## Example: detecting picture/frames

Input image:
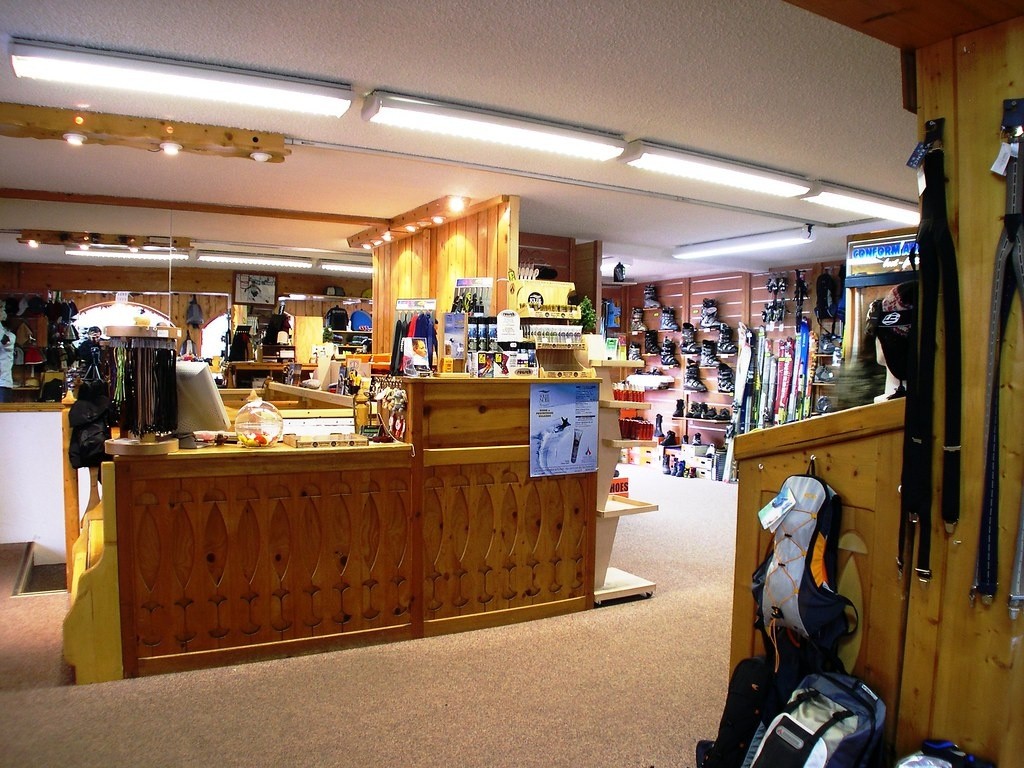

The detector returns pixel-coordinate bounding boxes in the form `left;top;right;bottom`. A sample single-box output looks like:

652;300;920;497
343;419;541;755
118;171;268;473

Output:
232;270;278;309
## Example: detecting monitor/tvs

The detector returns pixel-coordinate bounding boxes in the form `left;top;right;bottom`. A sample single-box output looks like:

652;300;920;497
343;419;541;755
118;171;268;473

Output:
173;361;231;448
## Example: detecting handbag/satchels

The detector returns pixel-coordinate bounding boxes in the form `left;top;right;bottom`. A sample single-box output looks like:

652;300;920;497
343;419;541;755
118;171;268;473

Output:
751;459;858;673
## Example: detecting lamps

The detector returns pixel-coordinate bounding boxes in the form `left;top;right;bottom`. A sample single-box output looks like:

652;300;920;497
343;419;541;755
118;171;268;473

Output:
9;36;351;121
61;133;90;146
129;246;142;253
360;88;623;162
250;152;272;163
28;241;41;249
799;180;920;225
78;243;90;250
158;142;182;157
362;216;445;250
672;225;816;259
617;139;811;200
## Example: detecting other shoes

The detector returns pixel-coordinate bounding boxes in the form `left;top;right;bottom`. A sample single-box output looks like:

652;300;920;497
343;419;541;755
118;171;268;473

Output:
662;454;696;478
692;433;701;445
685;402;730;420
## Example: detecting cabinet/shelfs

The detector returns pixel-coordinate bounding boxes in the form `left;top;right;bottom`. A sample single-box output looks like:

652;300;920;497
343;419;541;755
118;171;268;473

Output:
628;328;836;423
588;359;657;604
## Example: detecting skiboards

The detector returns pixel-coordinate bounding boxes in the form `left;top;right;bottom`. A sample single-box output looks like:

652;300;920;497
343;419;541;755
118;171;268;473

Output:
720;317;820;484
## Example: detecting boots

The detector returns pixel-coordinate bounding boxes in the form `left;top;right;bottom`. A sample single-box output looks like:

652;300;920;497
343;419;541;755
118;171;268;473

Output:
700;339;721;367
660;307;679;330
660;430;676;446
645;330;662;354
630;307;648;331
700;298;721;328
717;363;735;393
717;324;738;353
628;344;642;361
660;339;679;365
654;414;665;437
644;283;661;308
680;323;701;353
673;399;684;417
684;358;707;391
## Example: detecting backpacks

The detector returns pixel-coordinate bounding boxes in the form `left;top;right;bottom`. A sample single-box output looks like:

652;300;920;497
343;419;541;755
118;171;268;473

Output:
814;269;841;339
739;668;887;768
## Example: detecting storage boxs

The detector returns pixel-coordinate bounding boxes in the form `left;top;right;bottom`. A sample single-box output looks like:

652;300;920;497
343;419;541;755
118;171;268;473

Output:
621;438;736;483
508;279;575;312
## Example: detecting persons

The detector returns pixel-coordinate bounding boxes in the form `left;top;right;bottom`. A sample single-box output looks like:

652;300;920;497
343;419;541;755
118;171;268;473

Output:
77;326;108;378
411;339;427;365
0;324;16;402
